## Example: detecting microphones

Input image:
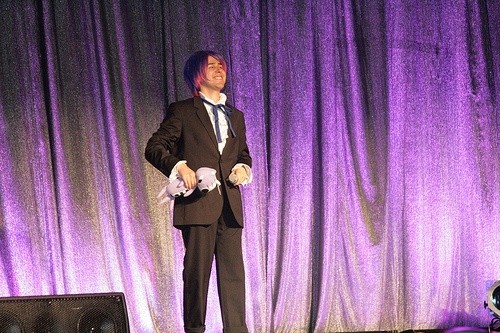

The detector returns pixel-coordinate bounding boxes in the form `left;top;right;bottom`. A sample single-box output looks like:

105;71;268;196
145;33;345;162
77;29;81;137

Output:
229;173;238;184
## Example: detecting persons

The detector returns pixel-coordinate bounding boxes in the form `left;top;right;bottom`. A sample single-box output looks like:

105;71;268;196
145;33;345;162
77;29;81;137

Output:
144;51;253;333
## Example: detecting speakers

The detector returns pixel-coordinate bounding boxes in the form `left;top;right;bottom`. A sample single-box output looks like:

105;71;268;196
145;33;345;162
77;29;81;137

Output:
0;292;130;333
